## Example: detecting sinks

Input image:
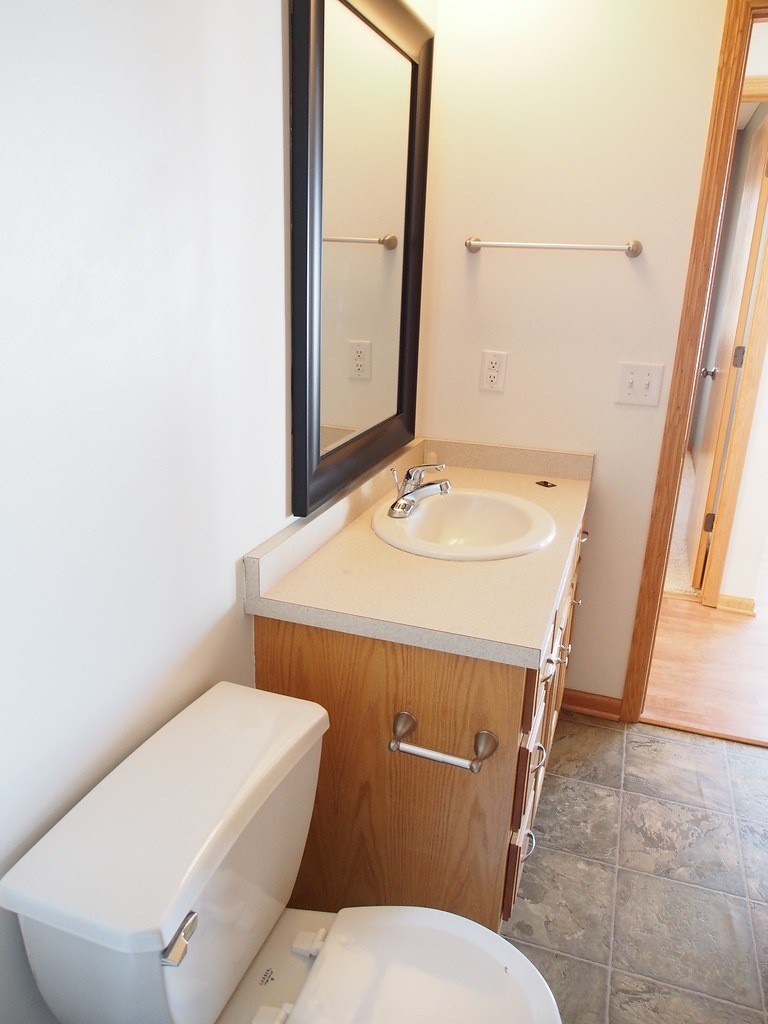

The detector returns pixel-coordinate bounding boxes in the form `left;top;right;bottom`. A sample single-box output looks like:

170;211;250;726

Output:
370;490;558;563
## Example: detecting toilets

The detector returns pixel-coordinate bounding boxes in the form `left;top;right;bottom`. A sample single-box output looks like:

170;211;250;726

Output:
3;680;562;1024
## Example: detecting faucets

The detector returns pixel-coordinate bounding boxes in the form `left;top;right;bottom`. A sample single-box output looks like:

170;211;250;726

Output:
387;462;453;518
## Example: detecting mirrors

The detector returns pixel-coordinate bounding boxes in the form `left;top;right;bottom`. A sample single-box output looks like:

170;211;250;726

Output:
293;0;434;516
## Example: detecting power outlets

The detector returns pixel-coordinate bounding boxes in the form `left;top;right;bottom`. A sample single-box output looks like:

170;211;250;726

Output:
479;349;507;392
348;341;372;379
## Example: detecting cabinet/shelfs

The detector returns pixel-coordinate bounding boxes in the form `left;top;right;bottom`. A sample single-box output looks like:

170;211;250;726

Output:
253;515;588;934
